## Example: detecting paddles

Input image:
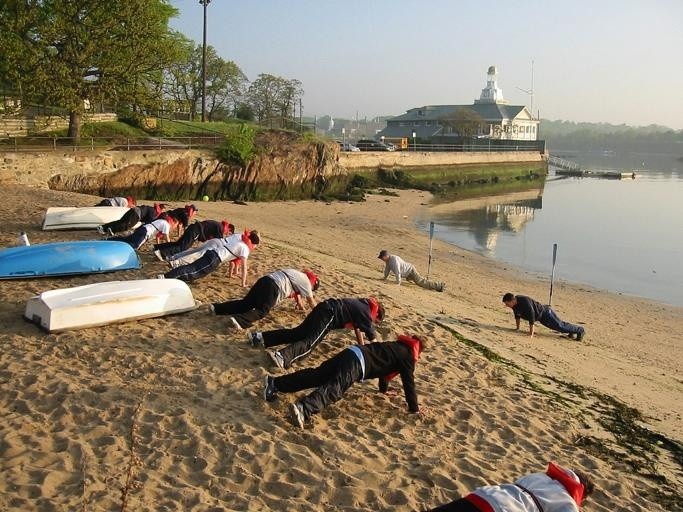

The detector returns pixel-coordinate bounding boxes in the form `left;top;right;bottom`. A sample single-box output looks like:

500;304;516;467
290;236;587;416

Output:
548;244;557;304
428;222;434;270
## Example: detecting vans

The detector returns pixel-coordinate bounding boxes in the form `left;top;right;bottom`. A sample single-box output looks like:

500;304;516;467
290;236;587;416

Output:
355;138;395;152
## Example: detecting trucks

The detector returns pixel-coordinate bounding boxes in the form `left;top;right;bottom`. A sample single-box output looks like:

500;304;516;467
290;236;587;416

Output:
378;136;408;151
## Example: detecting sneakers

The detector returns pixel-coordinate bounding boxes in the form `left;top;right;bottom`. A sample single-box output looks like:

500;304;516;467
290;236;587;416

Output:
289;400;306;429
568;326;585;341
377;250;391;258
230;316;242;329
440;281;446;292
97;225;179;279
267;351;284;368
207;303;216;313
264;373;277;403
247;332;260;347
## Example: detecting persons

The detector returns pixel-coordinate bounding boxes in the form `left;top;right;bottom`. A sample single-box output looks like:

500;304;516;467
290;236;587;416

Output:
209;269;319;331
83;97;91;113
94;196;260;288
263;335;429;430
248;297;385;371
429;462;593;512
378;250;445;292
502;293;586;341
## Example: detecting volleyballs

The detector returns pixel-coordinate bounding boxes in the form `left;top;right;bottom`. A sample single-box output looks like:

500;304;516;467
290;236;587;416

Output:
203;195;209;201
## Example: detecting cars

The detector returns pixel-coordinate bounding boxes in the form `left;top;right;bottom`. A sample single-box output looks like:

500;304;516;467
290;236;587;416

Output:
339;143;361;152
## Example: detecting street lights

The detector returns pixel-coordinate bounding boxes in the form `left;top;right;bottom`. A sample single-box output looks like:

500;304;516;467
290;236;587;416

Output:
199;0;212;121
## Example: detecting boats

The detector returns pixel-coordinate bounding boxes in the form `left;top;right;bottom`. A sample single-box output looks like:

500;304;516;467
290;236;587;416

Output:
0;241;141;282
41;205;142;231
22;278;199;335
